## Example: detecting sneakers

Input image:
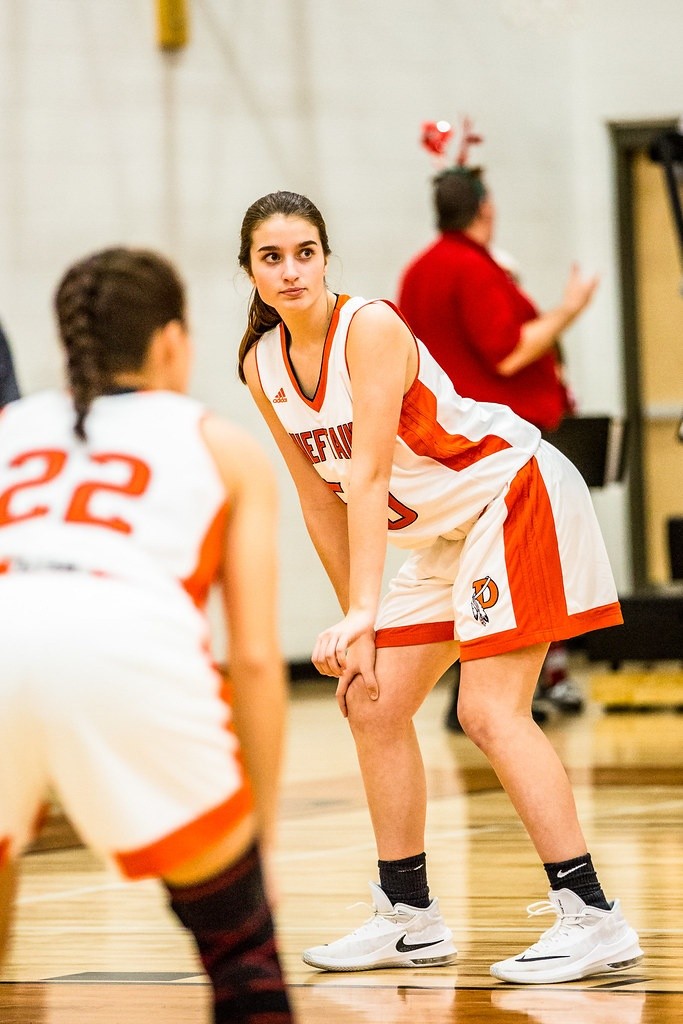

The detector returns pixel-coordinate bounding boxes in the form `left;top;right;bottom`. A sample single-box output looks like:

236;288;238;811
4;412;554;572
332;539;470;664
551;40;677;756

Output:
301;885;458;969
488;889;644;983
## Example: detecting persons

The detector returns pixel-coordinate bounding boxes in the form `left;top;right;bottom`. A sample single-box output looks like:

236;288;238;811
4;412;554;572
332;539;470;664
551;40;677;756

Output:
237;190;645;984
396;167;604;735
0;246;298;1024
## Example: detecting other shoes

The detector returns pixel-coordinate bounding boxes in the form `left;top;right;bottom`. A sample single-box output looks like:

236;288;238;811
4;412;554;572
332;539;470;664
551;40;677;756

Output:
449;653;583;732
